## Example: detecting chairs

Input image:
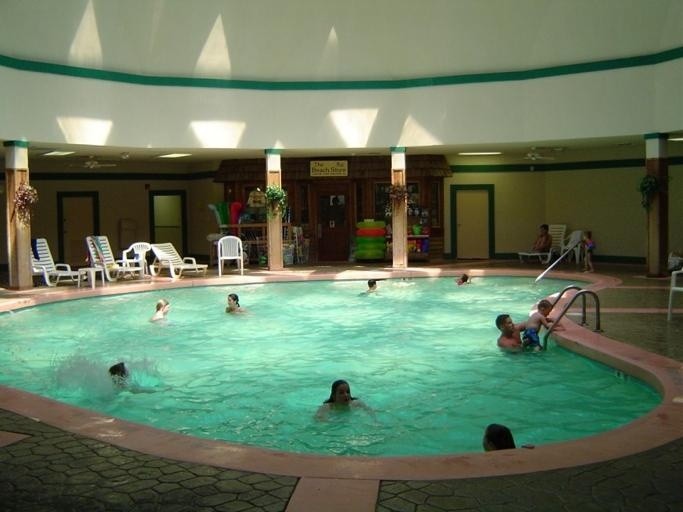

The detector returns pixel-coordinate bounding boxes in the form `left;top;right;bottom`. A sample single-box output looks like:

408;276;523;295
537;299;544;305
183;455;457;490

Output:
205;232;222;266
559;228;587;262
667;263;683;320
28;235;245;287
515;224;567;261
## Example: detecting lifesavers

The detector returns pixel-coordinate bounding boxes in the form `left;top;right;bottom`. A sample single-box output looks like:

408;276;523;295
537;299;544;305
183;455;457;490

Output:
354;220;386;260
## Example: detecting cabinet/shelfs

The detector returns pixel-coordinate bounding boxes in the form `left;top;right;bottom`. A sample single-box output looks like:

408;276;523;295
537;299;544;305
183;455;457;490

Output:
216;224;297;267
382;232;431;261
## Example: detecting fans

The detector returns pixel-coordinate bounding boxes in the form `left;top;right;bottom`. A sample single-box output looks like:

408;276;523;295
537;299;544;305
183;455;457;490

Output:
64;155;117;169
508;146;557;163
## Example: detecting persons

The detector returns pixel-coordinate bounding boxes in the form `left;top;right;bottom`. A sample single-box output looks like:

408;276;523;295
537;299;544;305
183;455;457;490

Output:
521;299;566;353
454;273;472;286
363;278;380;294
532;223;552;253
312;378;378;424
580;229;596;274
108;362;130;385
225;293;249;315
481;422;535;451
150;298;170;322
494;312;556;350
